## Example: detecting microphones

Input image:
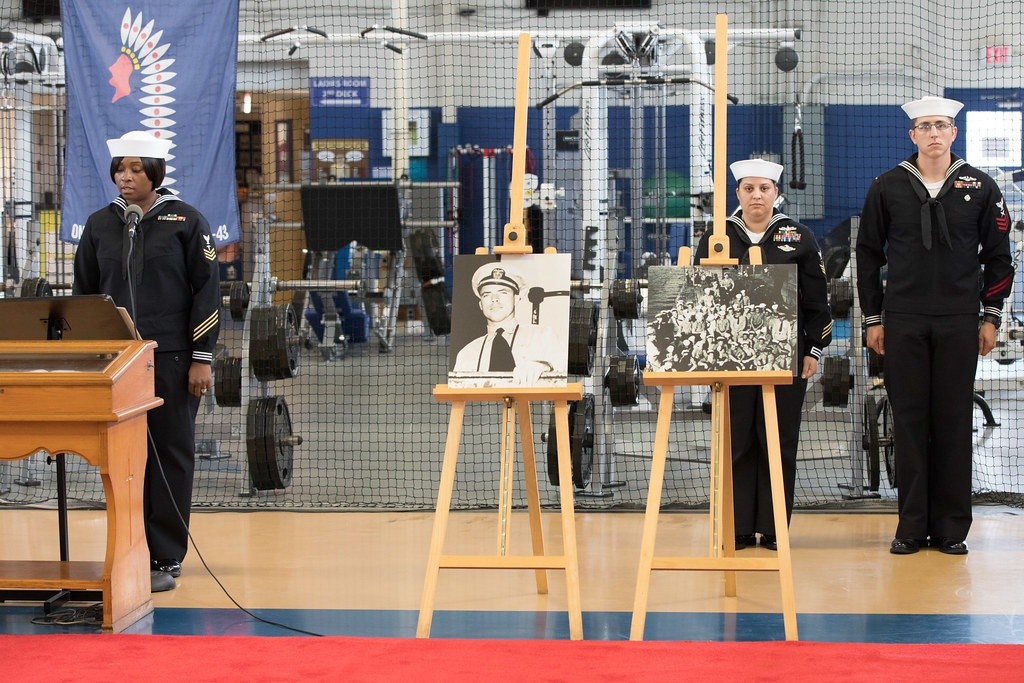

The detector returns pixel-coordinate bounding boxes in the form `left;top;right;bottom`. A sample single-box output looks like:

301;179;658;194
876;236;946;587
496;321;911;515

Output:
124;204;143;238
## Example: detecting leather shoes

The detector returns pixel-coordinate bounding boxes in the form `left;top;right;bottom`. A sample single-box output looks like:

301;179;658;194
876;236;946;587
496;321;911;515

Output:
890;536;923;554
938;538;968;554
151;558;182;577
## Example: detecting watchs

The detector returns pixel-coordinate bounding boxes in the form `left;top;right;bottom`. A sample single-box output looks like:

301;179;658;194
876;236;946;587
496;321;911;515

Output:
983;314;1001;329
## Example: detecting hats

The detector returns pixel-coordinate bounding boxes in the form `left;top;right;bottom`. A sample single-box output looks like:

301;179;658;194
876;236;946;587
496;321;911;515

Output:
470;261;524;295
900;96;964;119
729;159;784;184
105;130;172;158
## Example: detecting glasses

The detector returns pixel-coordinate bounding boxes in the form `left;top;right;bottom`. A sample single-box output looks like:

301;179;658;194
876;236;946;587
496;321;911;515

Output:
913;121;955;130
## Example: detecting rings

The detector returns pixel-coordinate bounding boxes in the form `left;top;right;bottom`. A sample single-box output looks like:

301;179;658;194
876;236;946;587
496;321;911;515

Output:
201;388;207;394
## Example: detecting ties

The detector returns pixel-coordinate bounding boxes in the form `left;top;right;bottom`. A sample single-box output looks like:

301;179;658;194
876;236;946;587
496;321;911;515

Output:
489;327;515;371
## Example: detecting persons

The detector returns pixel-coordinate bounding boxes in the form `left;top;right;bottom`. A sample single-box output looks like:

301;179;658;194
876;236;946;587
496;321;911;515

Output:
453;263;561;387
71;130;222;576
329;155;351;178
692;159;835;550
649;270;796;373
855;97;1016;555
239;167;260;199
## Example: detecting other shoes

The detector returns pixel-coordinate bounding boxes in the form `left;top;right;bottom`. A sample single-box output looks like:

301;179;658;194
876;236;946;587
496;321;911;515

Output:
735;531;756;550
759;534;778;550
